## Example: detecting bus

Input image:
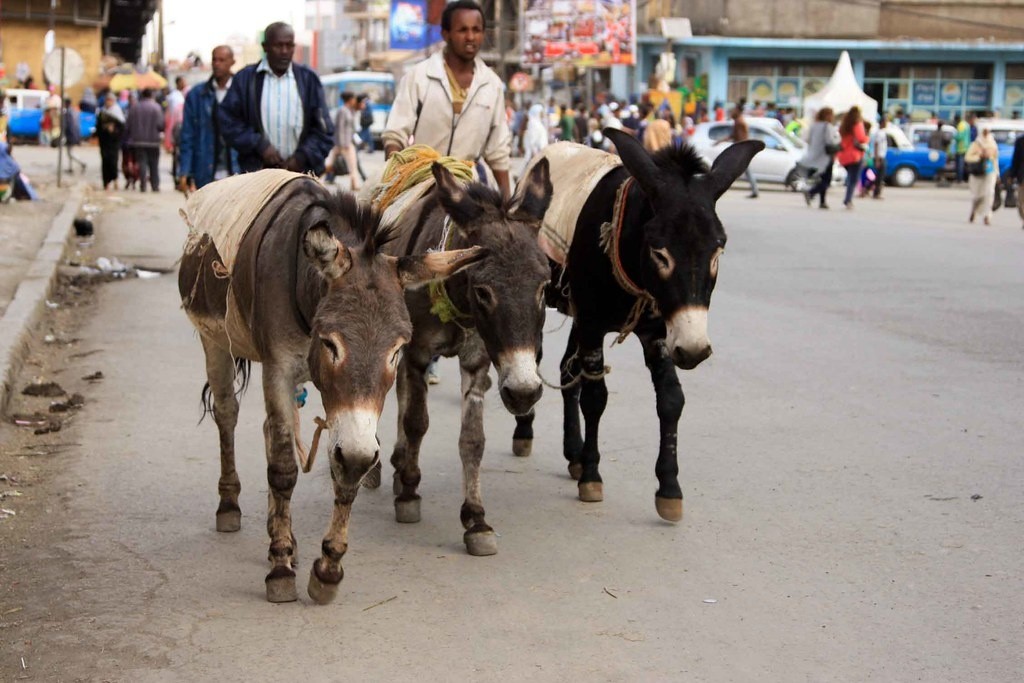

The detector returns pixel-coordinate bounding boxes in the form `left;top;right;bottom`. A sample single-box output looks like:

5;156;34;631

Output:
318;70;399;149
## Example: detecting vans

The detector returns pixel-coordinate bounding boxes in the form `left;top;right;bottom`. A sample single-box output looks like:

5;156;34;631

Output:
0;88;52;120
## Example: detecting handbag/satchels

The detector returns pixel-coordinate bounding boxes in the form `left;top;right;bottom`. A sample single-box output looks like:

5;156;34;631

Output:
360;115;373;127
1008;186;1016;209
992;185;1002;212
824;144;842;154
853;138;869;151
333;155;348;176
965;157;987;177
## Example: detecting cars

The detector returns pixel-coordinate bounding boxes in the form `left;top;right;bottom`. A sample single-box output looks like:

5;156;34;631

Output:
878;119;1024;189
682;122;848;192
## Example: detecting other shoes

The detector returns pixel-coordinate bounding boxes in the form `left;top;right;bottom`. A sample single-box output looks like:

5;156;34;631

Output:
804;191;810;205
820;204;828;208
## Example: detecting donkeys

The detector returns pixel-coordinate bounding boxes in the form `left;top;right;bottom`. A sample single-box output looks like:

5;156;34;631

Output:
178;126;766;604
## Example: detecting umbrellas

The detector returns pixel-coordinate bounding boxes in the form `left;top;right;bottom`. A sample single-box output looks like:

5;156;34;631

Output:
109;67;167;106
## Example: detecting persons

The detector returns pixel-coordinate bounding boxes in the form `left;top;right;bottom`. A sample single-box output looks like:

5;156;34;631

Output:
663;108;674;128
806;106;886;209
588;92;655;154
79;86;169;190
180;46;238;196
0;77;61;199
65;99;85;174
384;0;511;385
507;91;586;156
701;97;801;199
166;77;186;185
892;112;910;125
927;112;1024;228
326;94;366;182
324;91;361;189
360;94;374;153
217;22;333;174
681;118;693;129
